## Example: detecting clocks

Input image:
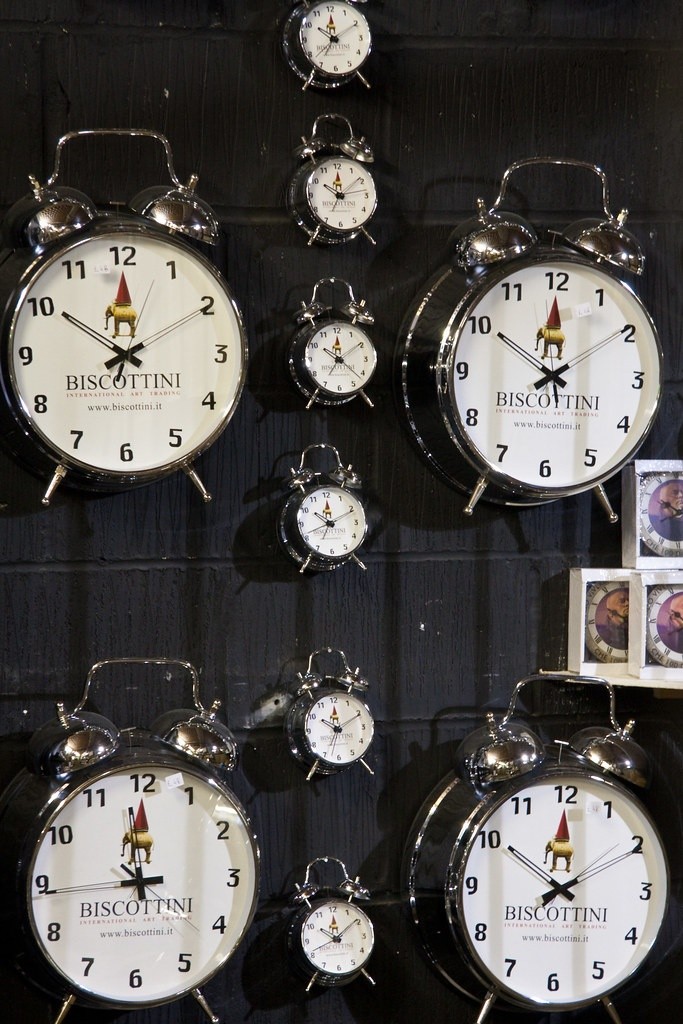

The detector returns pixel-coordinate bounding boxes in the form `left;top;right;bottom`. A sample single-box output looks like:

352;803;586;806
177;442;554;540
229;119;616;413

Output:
390;156;659;523
402;674;673;1024
0;128;250;503
274;442;370;574
284;113;379;245
288;277;378;410
285;856;377;991
0;656;261;1024
278;0;374;94
628;571;683;679
567;567;679;676
621;459;683;570
283;648;377;782
